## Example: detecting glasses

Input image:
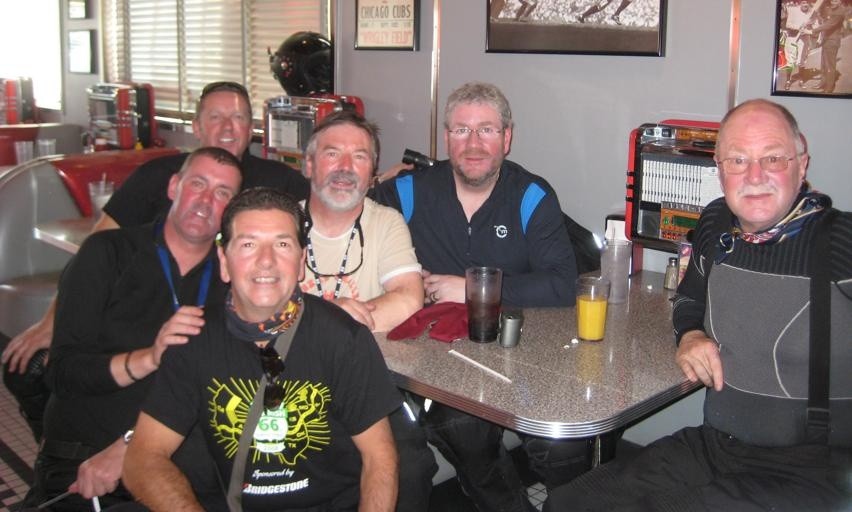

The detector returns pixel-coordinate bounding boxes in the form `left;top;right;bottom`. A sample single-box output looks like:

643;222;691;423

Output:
447;126;505;135
717;155;800;174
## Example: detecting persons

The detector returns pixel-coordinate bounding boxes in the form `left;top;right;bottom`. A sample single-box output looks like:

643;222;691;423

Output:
295;111;425;340
2;80;417;443
120;184;404;511
578;0;635;29
513;0;541;25
18;143;244;512
366;82;594;512
810;0;849;96
784;1;816;90
541;96;852;512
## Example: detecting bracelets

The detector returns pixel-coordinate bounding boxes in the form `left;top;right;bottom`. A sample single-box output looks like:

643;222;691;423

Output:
373;176;381;187
125;348;140;383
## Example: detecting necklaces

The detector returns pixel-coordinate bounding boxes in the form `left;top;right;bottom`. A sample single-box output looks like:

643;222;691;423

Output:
301;197;365;277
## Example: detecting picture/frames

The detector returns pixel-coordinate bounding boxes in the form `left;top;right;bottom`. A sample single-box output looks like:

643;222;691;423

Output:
67;0;94;74
354;0;420;51
770;0;852;99
485;0;667;57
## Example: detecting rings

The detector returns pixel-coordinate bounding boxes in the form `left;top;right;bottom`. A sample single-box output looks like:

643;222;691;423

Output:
430;290;437;305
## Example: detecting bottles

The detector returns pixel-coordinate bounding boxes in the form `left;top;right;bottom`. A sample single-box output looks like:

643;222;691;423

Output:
81;132;143;153
664;258;678;291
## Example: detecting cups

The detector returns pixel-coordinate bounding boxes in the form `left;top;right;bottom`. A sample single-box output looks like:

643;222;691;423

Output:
574;276;611;342
500;311;523;348
14;138;56;164
600;239;632;304
465;267;503;343
89;181;115;223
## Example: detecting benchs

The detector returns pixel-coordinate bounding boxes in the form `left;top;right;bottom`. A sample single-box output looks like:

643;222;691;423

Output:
1;148;196;342
